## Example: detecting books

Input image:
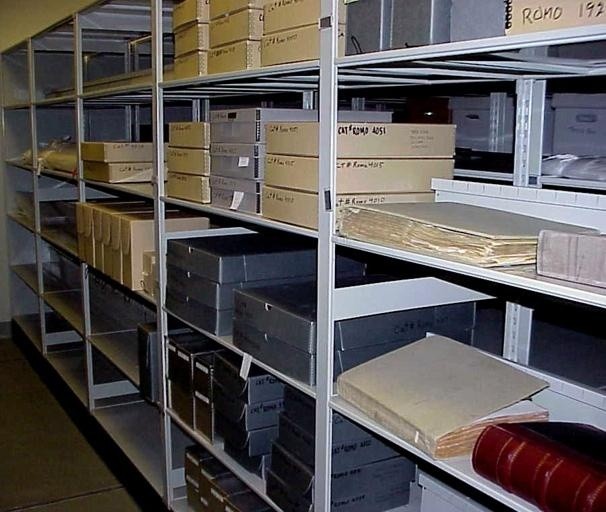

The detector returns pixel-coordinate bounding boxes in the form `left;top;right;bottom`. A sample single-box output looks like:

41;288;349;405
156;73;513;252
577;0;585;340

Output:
335;201;600;269
473;423;606;512
337;335;550;462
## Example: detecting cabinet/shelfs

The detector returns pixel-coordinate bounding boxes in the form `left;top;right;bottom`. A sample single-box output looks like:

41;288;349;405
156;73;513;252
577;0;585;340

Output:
30;5;198;434
153;6;526;511
0;2;605;512
74;3;365;512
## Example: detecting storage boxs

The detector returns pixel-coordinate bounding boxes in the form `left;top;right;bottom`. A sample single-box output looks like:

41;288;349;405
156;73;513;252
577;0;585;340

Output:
315;5;606;512
449;93;604;160
504;1;605;35
172;2;346;78
80;108;456;232
76;200;367;338
136;273;489;512
